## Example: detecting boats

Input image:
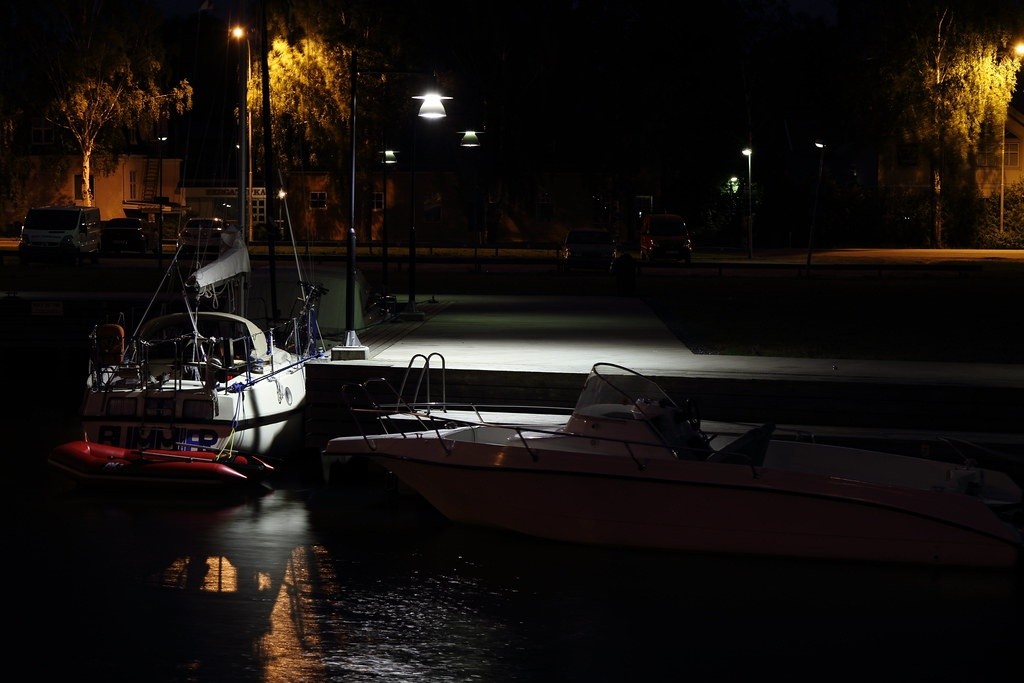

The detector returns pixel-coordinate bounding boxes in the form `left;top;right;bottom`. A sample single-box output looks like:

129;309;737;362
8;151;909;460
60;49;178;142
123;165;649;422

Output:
320;361;1023;578
72;213;341;458
349;351;1024;485
46;436;280;492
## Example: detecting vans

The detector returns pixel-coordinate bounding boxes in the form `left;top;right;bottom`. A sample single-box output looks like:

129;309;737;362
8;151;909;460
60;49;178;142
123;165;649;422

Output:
17;204;101;262
636;213;695;264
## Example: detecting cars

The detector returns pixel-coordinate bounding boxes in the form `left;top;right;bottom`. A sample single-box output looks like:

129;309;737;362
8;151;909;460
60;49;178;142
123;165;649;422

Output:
174;216;231;256
100;214;159;256
560;225;617;272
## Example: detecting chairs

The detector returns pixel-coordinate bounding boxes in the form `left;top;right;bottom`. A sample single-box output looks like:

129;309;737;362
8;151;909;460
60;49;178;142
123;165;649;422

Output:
708;423;776;464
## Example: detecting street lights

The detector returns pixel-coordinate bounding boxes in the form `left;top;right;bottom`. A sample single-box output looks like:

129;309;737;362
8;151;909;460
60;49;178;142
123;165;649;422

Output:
229;22;256;244
156;132;168;256
743;143;754;259
456;98;486;271
377;123;401;303
405;72;455;314
997;37;1024;234
805;137;828;264
276;186;287;229
